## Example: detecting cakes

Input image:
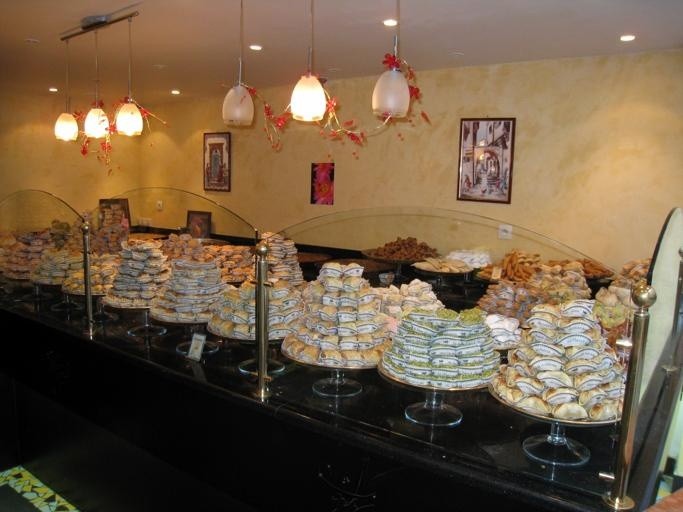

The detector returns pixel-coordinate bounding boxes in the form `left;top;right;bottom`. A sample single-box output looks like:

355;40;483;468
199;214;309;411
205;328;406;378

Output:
380;306;502;390
103;238;170;309
147;256;227;323
1;222;255;297
367;236;653;348
491;299;627;422
280;263;389;370
208;278;301;342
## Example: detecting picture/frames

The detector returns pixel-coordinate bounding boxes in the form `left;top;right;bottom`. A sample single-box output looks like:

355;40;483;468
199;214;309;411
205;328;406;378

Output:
454;116;516;204
202;131;231;192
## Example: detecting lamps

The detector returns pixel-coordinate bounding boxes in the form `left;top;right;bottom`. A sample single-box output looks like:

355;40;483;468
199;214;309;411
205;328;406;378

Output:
52;10;146;143
219;1;410;128
